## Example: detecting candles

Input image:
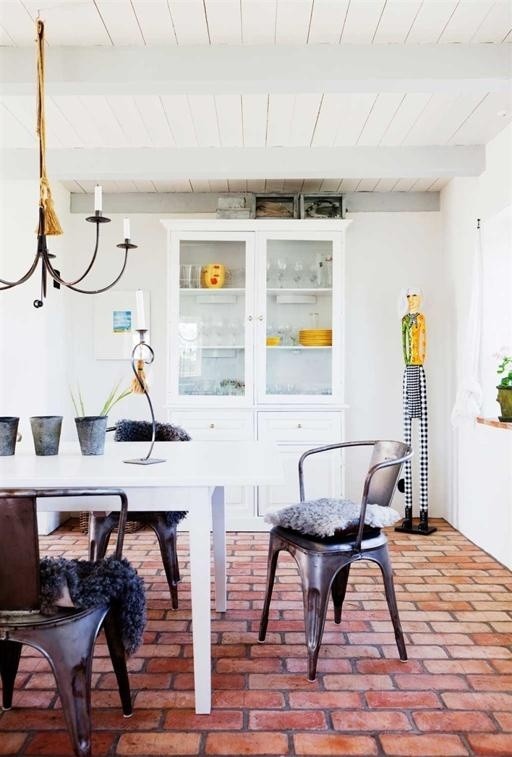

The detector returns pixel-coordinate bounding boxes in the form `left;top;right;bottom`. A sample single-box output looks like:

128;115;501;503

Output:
135;286;146;329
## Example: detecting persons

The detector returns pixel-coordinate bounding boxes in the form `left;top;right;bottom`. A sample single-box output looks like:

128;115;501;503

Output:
399;285;429;530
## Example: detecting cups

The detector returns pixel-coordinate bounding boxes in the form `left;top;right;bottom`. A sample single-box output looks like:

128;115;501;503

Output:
0;416;20;456
265;380;333;395
179;379;243;396
179;262;203;289
75;414;109;455
30;414;62;456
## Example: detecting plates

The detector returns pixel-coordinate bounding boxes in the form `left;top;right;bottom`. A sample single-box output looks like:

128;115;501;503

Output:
298;328;334;347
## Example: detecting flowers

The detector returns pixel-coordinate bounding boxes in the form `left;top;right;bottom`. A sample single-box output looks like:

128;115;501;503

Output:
496;355;512;387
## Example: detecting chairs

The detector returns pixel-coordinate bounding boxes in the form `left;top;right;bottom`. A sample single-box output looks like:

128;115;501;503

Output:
258;438;416;683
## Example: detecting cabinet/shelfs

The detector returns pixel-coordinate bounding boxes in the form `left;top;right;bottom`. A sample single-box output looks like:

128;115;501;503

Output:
165;230;351;532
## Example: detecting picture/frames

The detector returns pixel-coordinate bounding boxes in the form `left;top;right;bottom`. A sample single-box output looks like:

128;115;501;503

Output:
252;192;346;219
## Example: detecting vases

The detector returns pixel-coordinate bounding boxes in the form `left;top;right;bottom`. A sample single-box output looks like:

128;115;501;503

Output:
494;385;512;423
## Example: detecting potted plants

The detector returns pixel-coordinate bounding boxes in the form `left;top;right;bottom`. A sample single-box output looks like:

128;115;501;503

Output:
67;379;134;457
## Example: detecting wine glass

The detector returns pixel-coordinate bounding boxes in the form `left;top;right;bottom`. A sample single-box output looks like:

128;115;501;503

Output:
266;253;332;288
266;321;301;345
177;317;244;348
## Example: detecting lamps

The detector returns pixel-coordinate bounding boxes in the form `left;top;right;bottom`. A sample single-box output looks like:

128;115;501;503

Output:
2;15;140;308
